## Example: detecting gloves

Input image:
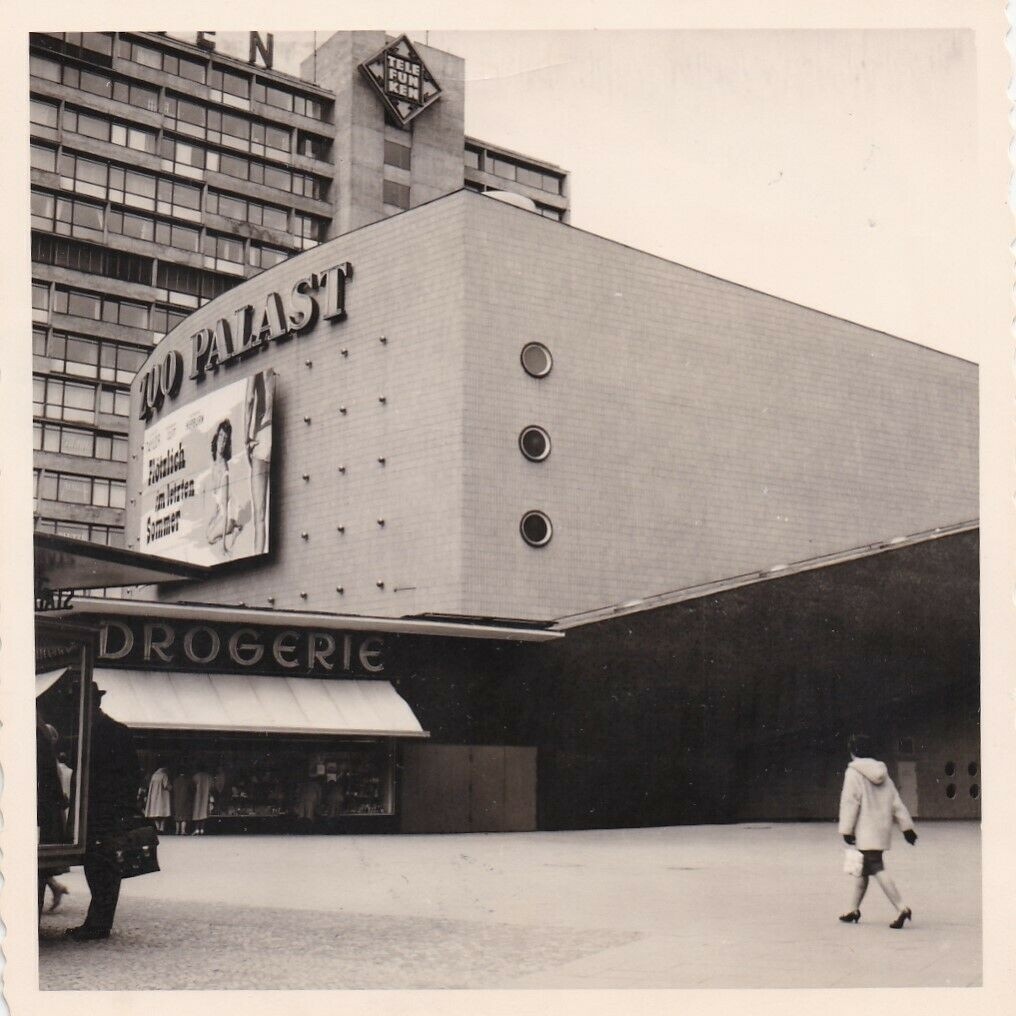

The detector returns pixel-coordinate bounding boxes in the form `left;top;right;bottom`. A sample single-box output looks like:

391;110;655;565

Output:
903;829;917;845
843;834;856;845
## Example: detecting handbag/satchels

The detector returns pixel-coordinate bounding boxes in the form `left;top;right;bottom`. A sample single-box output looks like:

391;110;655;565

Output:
839;847;864;877
108;825;160;879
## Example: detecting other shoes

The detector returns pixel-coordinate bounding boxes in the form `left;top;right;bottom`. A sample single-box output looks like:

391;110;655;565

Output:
180;831;189;835
175;831;179;835
156;830;163;835
65;925;111;941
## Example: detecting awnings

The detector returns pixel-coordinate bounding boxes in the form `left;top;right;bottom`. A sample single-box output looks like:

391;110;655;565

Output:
91;669;429;737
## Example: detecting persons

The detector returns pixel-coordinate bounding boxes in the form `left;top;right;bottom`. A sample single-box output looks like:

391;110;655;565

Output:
42;702;139;940
839;735;918;929
144;761;211;836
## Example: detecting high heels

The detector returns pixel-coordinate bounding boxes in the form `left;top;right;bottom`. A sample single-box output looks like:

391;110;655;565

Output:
192;829;199;836
200;828;204;835
48;884;69;912
889;906;911;929
839;909;860;924
